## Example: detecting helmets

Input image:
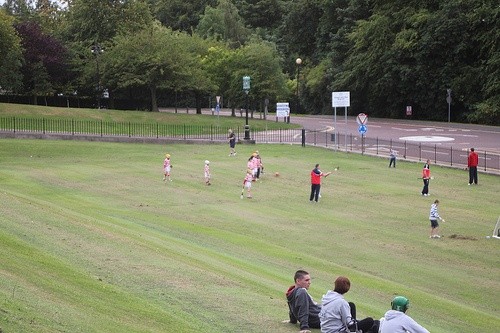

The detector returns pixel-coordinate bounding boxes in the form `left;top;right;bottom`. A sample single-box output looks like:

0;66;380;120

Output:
390;296;410;314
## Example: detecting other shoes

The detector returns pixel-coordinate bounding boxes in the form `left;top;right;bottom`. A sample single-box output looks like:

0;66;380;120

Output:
247;195;252;197
206;181;211;184
468;181;476;184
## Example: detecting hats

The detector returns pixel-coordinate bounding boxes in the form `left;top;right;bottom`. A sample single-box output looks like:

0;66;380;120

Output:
205;159;209;164
256;150;259;155
253;152;257;156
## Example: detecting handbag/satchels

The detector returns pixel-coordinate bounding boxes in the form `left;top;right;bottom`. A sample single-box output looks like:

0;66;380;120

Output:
336;316;363;332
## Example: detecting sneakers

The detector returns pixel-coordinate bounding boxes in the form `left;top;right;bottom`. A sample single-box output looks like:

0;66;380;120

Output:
430;234;440;238
234;151;237;155
422;192;430;196
228;154;232;156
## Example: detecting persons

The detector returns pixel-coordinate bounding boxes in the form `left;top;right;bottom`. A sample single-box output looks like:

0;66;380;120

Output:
205;160;212;185
240;151;264;199
162;153;173;182
378;296;430;333
318;276;374;333
389;148;396;168
309;164;327;203
468;148;479;185
420;160;431;196
228;128;237;157
282;270;356;333
429;200;445;238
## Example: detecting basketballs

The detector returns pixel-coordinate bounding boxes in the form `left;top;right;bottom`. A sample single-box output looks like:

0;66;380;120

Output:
275;171;280;179
